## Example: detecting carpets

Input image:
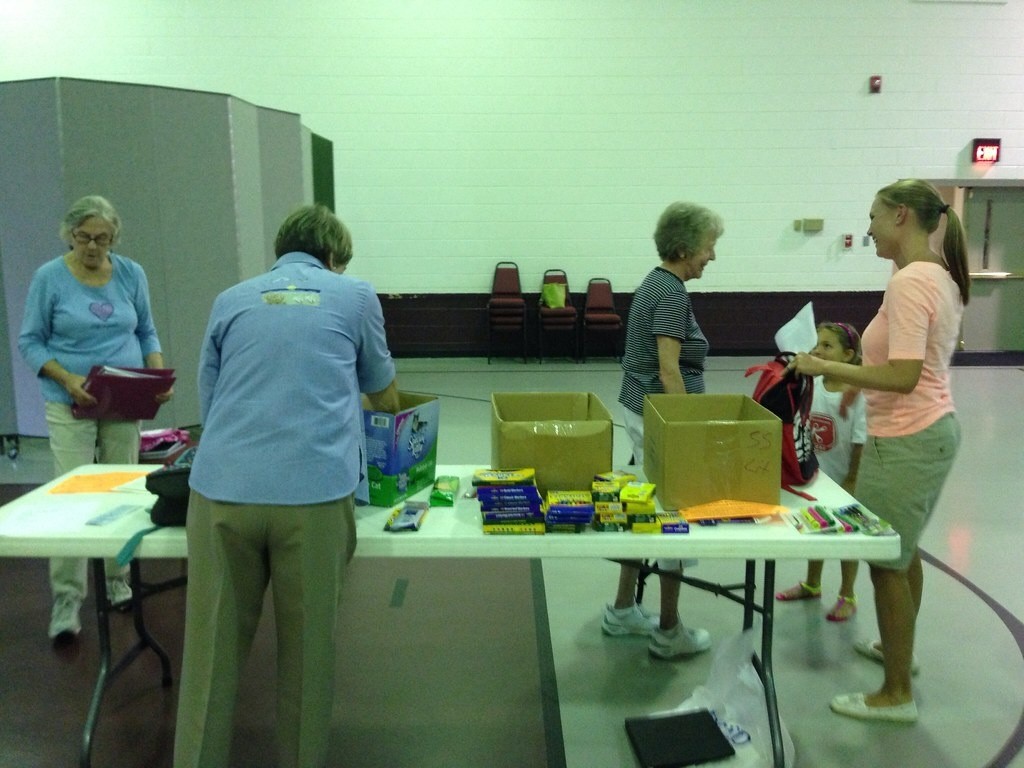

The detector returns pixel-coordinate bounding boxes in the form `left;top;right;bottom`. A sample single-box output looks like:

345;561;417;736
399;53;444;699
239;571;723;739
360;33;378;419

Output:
0;482;566;768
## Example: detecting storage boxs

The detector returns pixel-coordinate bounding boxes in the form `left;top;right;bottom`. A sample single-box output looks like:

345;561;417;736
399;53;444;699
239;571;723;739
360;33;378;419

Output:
643;394;783;510
490;391;613;495
360;390;441;507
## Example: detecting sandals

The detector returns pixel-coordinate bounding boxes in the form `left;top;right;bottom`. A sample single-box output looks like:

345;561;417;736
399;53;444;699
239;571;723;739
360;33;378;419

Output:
826;592;857;622
775;582;822;602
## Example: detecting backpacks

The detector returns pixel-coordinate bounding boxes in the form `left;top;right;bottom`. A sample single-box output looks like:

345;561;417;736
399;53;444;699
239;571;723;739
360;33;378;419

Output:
744;352;821;501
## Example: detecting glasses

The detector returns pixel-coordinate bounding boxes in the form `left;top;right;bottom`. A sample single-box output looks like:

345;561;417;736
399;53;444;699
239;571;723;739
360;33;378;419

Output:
71;228;113;245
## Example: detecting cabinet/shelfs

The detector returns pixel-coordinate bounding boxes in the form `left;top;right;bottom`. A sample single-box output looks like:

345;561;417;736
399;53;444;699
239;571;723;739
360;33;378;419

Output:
902;177;1024;353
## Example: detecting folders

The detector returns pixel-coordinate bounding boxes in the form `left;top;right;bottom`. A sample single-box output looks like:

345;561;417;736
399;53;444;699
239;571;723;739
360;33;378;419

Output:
71;363;176;420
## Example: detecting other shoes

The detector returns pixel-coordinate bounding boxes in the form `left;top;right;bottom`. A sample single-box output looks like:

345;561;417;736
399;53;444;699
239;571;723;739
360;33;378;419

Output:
830;692;919;723
854;639;919;674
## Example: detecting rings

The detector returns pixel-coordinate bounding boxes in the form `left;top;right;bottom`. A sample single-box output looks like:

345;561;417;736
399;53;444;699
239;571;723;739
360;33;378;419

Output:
786;363;792;372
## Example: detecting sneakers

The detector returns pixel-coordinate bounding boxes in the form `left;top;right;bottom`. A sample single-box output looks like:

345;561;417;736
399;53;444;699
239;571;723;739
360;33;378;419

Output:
48;595;81;640
601;603;661;636
647;613;714;660
106;577;132;606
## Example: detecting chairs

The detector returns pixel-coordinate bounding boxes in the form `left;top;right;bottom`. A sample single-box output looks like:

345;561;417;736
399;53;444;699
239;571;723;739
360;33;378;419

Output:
486;261;528;365
536;268;580;365
581;277;625;364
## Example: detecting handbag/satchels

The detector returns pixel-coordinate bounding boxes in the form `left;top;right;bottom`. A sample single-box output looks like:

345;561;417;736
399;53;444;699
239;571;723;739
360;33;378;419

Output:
543;282;567;308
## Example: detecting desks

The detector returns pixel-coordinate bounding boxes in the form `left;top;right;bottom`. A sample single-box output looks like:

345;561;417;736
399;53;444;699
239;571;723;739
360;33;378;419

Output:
0;467;900;768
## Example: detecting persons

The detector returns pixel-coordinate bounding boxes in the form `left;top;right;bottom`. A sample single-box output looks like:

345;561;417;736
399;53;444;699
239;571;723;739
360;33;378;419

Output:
601;201;725;658
781;181;970;722
17;195;174;653
777;321;867;620
173;208;398;768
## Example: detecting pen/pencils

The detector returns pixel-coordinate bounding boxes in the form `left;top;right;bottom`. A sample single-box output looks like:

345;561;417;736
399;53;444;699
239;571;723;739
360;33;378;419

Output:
786;503;882;534
